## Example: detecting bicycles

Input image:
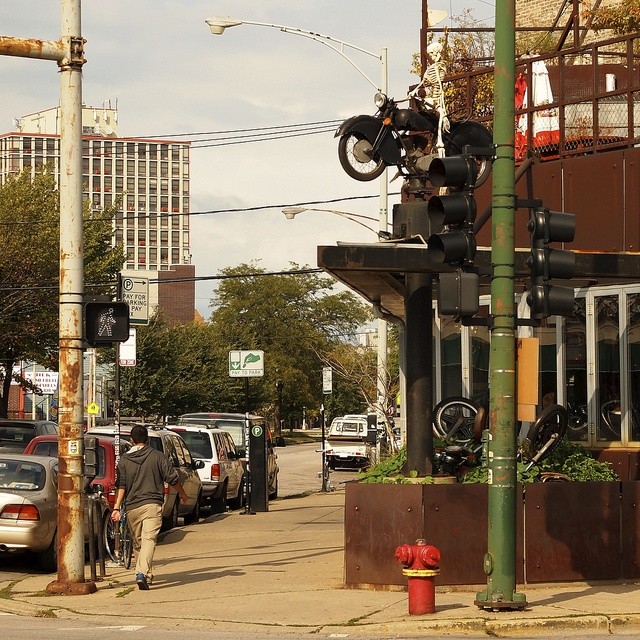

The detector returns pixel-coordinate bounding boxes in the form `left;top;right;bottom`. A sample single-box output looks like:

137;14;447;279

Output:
106;512;133;566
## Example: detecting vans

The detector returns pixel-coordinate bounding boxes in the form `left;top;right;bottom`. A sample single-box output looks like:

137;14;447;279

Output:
88;427;204;525
166;427;244;508
181;411;283;498
27;436;133;511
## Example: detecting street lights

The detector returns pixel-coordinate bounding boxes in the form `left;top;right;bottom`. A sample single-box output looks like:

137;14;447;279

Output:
283;205;389;415
205;18;388;238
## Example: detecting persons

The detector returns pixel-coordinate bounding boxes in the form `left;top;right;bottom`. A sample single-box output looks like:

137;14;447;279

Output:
111;426;189;590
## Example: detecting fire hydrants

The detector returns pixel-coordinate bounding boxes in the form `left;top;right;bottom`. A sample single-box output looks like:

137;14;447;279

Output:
395;538;441;615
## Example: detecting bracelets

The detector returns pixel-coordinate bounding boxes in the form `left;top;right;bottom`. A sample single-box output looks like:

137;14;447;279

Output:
113;509;120;512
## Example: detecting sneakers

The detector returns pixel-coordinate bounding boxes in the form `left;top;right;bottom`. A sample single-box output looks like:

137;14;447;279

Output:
135;573;149;591
144;571;154;583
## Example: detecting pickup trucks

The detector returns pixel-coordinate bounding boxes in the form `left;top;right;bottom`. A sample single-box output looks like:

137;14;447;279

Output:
324;416;368;470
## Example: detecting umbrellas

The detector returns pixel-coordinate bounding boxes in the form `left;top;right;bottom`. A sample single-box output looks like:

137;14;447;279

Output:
517;51;559;148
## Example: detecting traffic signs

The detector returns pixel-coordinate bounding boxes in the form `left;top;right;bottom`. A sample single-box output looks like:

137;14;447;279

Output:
124;278;149;325
229;350;264;378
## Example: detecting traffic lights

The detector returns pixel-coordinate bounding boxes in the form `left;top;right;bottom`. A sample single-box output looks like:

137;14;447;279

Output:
85;305;129;341
429;146;475;272
530;210;575;317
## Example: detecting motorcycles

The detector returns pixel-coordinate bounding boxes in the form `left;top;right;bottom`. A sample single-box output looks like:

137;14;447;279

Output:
433;398;568;473
378;418;400;448
333;90;490;189
568;401;637;440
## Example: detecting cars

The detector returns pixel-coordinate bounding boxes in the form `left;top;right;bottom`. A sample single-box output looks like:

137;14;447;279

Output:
0;455;59;570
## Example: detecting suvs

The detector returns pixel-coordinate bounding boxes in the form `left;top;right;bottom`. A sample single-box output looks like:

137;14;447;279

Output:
1;419;55;451
